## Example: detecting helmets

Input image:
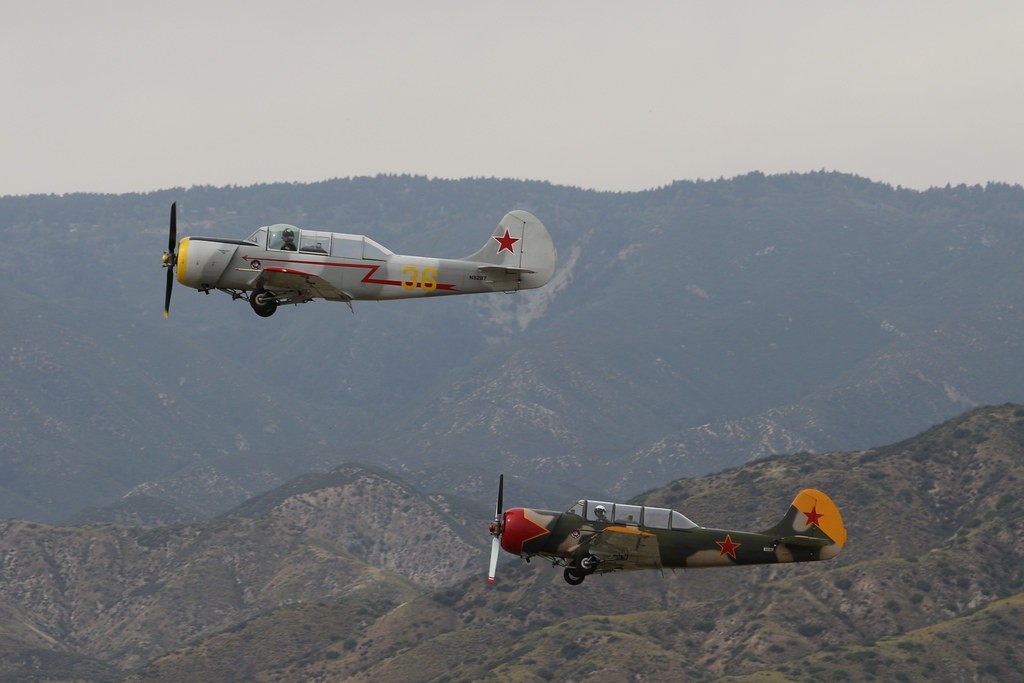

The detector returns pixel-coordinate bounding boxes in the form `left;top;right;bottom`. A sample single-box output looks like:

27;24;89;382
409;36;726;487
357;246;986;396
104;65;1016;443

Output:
282;228;294;243
594;506;606;516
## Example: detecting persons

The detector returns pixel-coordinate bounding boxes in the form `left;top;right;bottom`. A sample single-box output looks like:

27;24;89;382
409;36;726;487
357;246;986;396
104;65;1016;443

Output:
594;505;610;522
281;228;297;251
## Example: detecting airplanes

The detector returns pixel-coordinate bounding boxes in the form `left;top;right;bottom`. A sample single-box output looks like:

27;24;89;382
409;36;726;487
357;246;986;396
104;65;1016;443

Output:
488;474;847;585
163;202;555;320
